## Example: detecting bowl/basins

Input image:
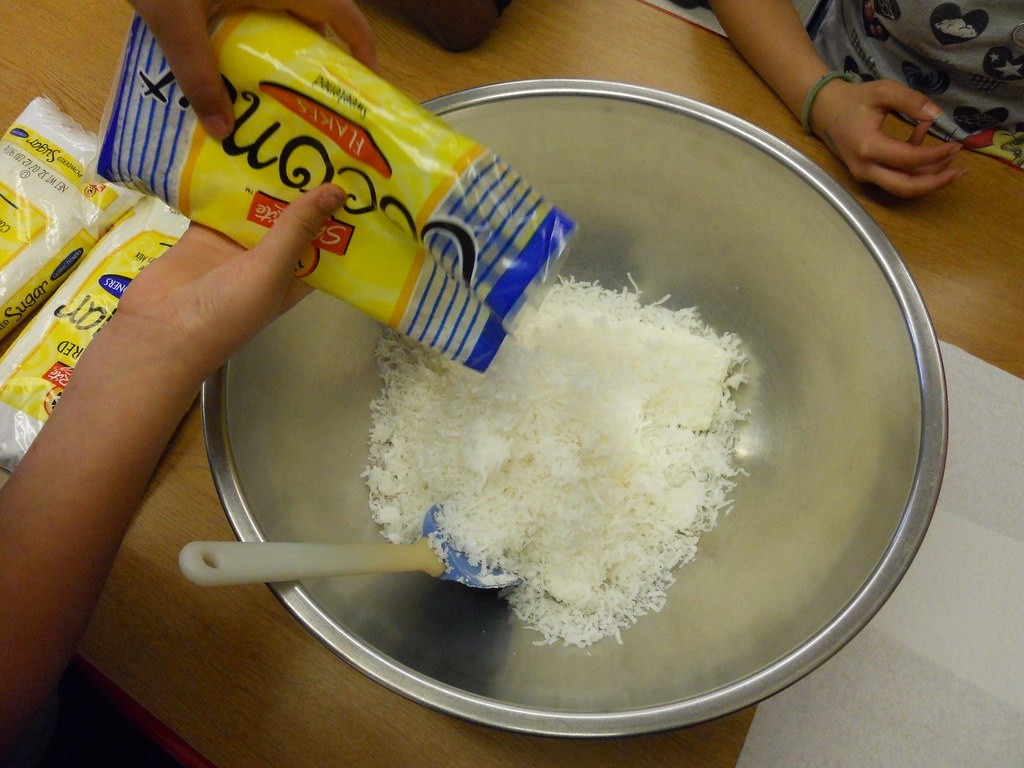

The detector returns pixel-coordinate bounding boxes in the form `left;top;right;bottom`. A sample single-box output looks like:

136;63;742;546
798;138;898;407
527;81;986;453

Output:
197;76;947;737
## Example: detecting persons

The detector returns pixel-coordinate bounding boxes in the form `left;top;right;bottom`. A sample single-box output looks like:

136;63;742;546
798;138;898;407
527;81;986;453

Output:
1;0;388;767
398;0;512;52
670;0;1023;199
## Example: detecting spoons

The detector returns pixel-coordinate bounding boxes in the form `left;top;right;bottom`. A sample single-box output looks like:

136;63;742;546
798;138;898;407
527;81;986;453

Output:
181;509;533;590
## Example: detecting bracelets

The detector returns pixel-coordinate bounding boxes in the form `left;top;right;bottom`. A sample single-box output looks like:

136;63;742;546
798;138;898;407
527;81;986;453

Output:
802;71;853;134
